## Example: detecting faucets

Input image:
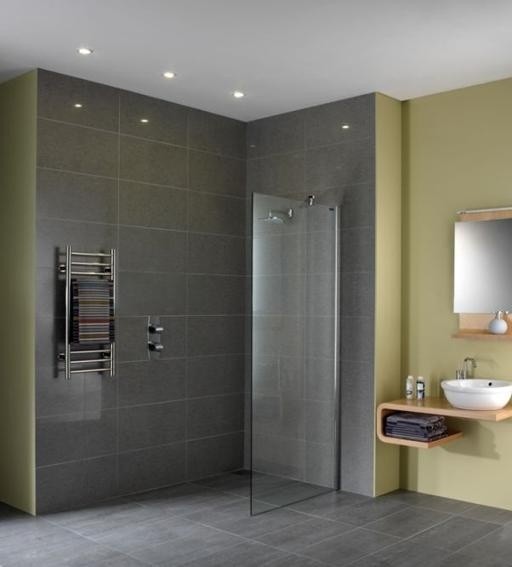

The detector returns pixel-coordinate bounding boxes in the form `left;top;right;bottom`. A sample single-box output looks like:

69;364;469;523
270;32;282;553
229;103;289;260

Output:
461;357;478;378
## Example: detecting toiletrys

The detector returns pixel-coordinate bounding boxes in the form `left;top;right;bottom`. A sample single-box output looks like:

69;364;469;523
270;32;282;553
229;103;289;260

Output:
488;311;508;333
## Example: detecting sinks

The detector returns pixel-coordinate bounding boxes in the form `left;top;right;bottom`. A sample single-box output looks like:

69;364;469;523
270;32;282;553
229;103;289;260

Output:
440;378;512;410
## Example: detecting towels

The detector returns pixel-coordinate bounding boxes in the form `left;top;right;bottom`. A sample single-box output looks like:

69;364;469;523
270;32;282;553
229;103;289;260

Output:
385;409;450;445
71;278;115;345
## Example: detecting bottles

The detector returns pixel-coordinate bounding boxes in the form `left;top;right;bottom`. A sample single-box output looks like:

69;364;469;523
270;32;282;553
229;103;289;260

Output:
405;374;425;400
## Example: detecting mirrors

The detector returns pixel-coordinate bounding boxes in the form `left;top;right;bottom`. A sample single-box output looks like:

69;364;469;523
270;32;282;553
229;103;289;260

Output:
452;205;512;339
